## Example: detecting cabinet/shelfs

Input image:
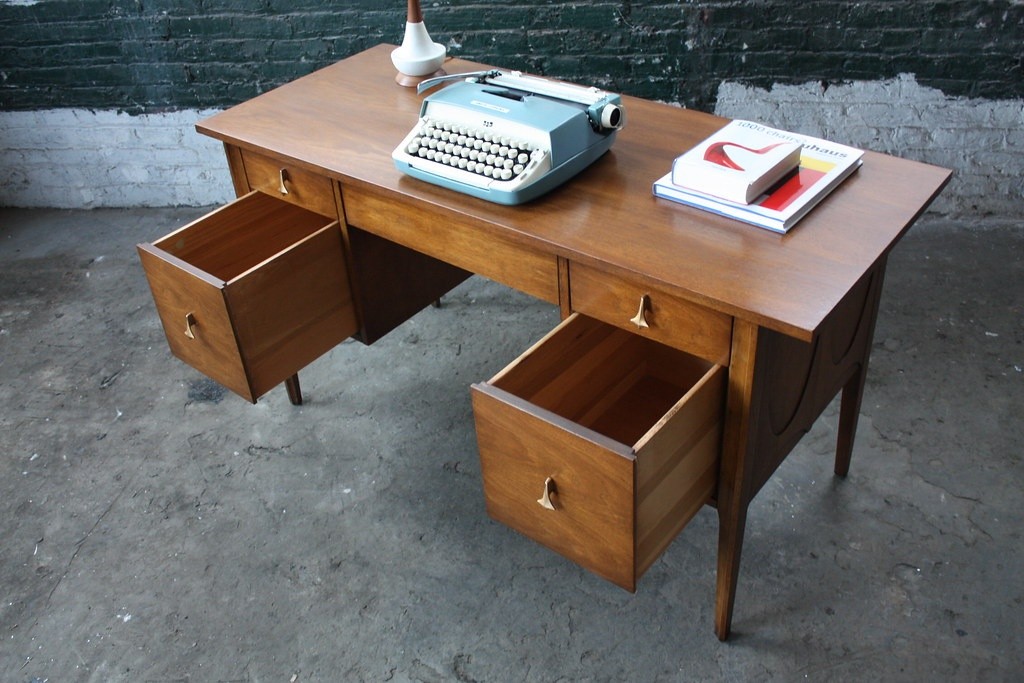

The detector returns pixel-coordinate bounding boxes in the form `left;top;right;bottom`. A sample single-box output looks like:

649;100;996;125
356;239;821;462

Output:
134;43;956;645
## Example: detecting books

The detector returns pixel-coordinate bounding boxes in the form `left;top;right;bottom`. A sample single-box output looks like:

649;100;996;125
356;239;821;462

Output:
652;115;867;236
671;117;801;208
